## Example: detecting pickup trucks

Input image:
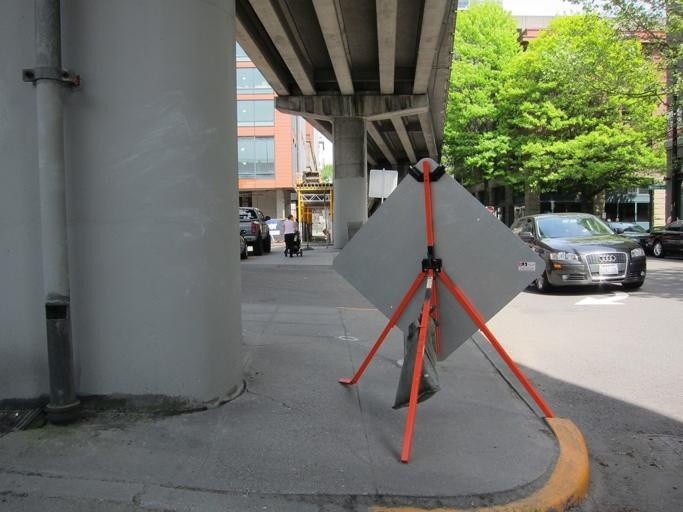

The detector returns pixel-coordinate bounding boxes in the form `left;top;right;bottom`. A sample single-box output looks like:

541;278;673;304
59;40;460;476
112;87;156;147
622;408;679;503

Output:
239;207;271;255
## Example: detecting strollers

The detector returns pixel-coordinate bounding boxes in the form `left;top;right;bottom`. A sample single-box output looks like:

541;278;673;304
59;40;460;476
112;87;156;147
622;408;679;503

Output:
283;232;303;257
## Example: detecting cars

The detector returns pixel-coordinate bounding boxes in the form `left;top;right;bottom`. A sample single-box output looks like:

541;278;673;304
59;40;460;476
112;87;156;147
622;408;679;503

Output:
239;230;249;261
509;211;682;294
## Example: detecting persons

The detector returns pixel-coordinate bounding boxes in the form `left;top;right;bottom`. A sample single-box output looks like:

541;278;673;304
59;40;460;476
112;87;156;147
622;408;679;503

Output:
281;215;298;257
